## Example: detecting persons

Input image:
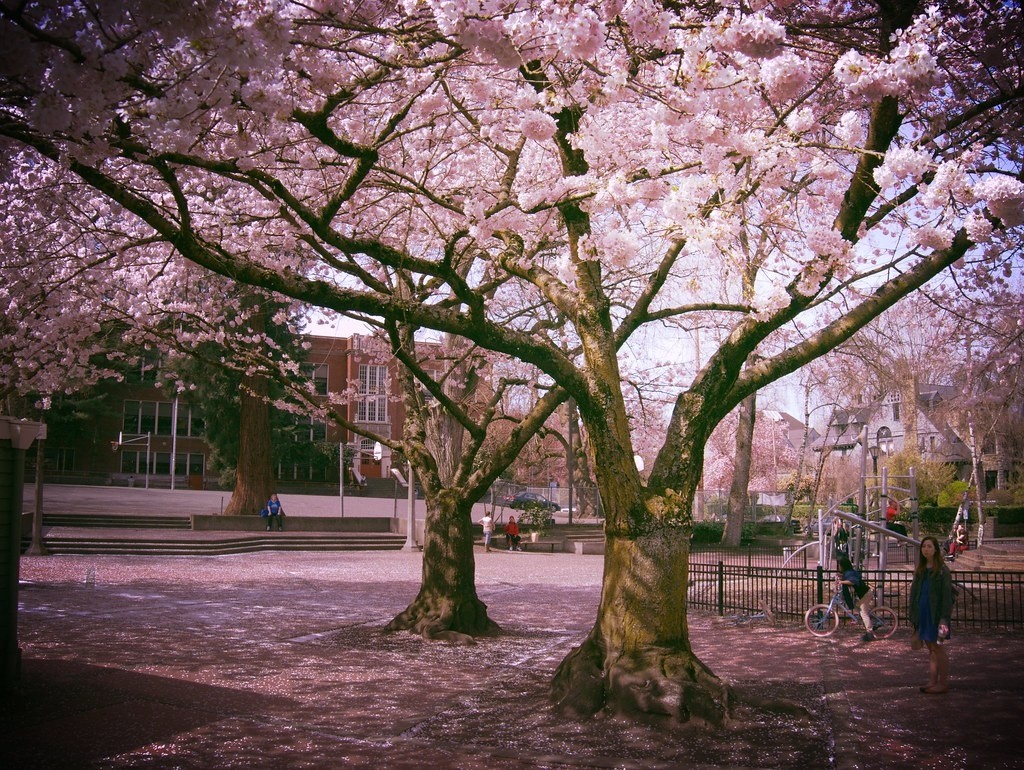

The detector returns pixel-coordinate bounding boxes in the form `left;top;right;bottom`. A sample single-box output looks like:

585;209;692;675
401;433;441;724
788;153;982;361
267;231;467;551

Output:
832;523;856;576
886;499;909;547
482;511;495;552
910;536;954;693
503;516;520;551
349;473;367;496
265;494;283;531
838;558;877;642
943;522;969;561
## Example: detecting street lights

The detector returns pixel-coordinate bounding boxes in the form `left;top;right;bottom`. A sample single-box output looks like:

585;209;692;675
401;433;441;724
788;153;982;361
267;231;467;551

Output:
868;446;882;558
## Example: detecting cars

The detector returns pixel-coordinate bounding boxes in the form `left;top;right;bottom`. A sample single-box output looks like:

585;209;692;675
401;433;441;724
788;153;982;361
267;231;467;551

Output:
504;490;562;515
756;514;801;536
802;516;851;537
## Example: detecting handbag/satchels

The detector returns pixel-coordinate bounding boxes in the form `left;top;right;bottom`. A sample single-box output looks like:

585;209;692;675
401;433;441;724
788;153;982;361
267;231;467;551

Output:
887;520;895;528
261;509;268;518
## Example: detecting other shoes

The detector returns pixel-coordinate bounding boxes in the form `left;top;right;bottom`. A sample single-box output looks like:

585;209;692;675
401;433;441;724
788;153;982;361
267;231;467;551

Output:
944;557;954;562
516;546;522;551
485;546;491;552
267;526;271;531
944;554;953;559
279;527;283;531
860;633;875;642
509;546;514;551
919;684;949;693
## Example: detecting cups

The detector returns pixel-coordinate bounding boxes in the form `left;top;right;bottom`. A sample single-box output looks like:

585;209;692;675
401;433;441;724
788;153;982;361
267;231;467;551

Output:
937;629;946;645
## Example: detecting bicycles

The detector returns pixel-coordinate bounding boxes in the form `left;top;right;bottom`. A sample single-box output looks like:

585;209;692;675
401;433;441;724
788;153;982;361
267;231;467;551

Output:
804;575;898;638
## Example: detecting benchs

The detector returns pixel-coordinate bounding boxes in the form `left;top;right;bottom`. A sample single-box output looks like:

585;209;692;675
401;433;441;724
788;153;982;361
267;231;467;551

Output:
961;539;977;554
520;541;561;553
